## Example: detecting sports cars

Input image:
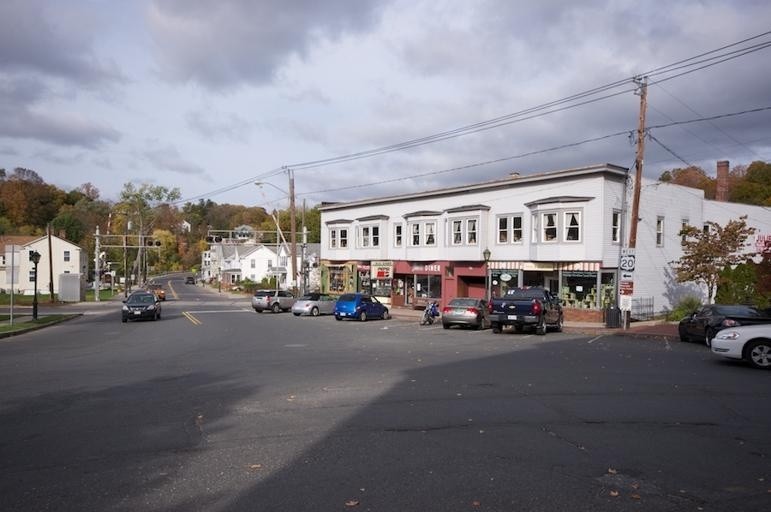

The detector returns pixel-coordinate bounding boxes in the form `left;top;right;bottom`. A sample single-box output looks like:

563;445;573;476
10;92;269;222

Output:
678;302;771;345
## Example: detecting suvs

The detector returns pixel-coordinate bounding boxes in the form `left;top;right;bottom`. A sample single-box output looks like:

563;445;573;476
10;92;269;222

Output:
251;290;298;314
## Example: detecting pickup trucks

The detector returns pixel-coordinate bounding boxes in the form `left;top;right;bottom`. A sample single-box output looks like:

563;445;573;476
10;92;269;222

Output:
490;284;563;335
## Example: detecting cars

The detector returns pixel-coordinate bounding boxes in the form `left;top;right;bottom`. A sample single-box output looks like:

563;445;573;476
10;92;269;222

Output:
291;292;338;317
185;276;196;285
145;283;168;300
121;292;163;323
332;294;390;322
441;297;490;329
709;323;770;369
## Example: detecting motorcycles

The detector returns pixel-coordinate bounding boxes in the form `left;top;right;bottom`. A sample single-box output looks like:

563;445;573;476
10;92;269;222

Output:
419;299;440;325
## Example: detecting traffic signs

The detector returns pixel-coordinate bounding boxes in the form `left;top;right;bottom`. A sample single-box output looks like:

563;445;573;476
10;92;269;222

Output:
620;249;635;281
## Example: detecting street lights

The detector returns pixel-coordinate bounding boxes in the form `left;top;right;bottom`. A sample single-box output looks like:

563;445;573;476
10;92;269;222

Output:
254;178;298;292
30;250;41;321
483;246;491;302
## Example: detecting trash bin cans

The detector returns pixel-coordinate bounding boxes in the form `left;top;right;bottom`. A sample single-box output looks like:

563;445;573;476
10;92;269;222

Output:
606;303;620;328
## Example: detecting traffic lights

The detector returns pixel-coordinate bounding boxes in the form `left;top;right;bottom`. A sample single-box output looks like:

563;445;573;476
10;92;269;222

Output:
148;240;160;246
206;237;223;243
